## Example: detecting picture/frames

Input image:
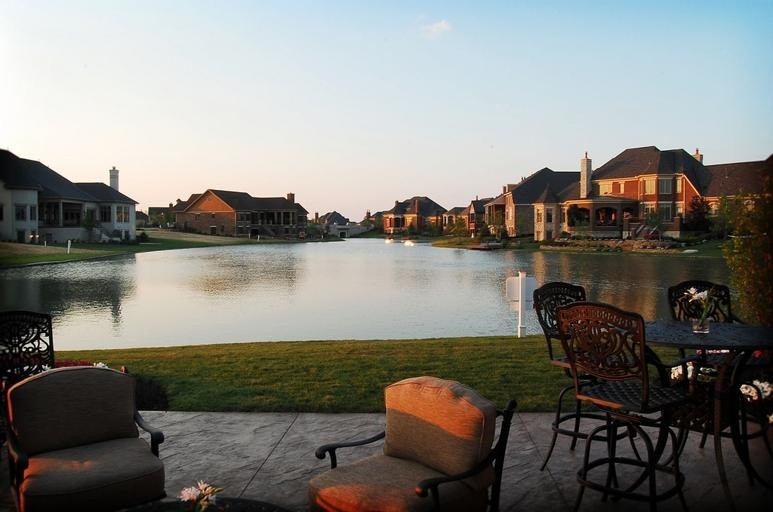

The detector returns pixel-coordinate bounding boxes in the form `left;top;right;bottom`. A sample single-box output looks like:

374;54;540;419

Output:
600;319;773;511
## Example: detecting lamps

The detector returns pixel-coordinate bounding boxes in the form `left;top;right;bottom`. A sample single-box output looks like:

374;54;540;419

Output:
667;280;749;470
555;300;691;512
533;283;642;489
309;375;517;512
0;310;57;463
0;365;167;512
699;363;773;488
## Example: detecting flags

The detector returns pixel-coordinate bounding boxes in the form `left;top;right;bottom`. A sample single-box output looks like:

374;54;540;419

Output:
691;318;711;333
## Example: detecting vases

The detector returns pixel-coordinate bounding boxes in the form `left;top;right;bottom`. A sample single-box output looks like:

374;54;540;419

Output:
176;479;224;512
684;285;718;326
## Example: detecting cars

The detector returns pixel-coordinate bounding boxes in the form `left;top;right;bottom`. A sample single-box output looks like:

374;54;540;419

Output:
692;317;714;334
